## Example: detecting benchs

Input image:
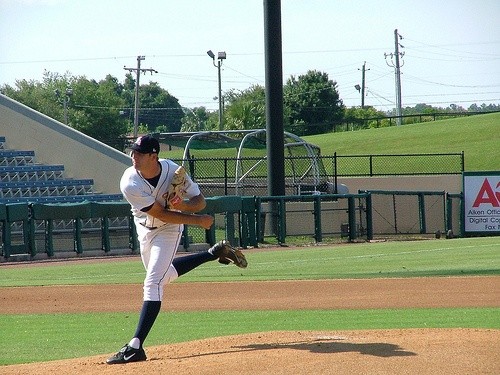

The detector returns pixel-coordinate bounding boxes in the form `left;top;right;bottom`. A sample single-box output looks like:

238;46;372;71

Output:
0;134;124;205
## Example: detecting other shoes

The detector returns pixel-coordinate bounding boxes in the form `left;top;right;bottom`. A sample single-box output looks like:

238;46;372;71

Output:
211;240;248;269
106;344;147;365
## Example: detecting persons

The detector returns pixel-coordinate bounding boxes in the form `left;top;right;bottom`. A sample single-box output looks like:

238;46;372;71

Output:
106;135;248;365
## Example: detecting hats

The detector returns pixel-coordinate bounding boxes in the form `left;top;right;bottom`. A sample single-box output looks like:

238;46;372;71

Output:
129;136;160;153
